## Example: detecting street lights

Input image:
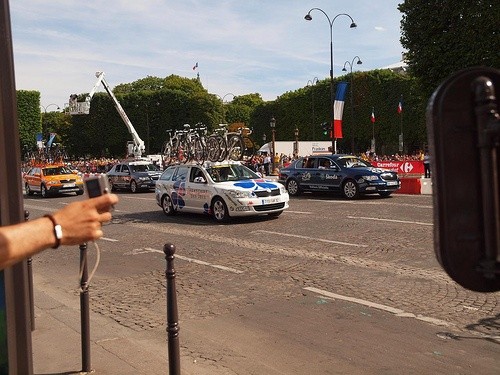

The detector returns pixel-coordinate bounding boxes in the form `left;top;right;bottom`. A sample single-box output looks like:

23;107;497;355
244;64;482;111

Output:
134;97;160;155
269;117;279;176
303;7;359;153
294;128;300;159
39;104;61;163
209;93;237;125
305;77;321;141
341;56;362;155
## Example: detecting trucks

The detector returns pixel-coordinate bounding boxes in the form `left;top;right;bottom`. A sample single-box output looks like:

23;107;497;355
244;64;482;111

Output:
255;140;337;159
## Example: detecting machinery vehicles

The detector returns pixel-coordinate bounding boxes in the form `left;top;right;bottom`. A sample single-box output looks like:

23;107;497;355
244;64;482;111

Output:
68;71;164;164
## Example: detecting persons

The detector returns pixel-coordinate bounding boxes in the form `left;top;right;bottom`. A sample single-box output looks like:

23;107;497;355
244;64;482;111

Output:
0;193;119;272
302;154;313;168
21;161;68;173
70;157;121;174
424;152;432;179
246;152;302;176
349;150;425;164
77;94;86;102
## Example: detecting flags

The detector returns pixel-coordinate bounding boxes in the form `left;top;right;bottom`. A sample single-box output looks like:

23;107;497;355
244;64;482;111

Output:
47;133;56;147
329;83;348;138
371;109;375;122
36;132;43;149
397;98;403;114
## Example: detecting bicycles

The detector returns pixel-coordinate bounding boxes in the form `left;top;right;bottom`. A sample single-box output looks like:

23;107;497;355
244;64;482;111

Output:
160;123;256;169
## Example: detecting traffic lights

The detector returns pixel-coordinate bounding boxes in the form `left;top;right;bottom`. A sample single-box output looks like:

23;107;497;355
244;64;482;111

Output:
321;121;329;137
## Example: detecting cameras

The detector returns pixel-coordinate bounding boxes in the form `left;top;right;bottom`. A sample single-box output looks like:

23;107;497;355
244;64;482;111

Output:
84;174;115;214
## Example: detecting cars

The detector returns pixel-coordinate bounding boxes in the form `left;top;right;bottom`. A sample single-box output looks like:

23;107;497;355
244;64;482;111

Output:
155;159;290;224
23;164;84;199
276;153;400;201
104;163;162;193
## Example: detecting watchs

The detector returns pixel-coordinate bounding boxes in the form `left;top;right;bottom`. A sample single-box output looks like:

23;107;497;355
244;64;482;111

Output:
43;213;63;250
